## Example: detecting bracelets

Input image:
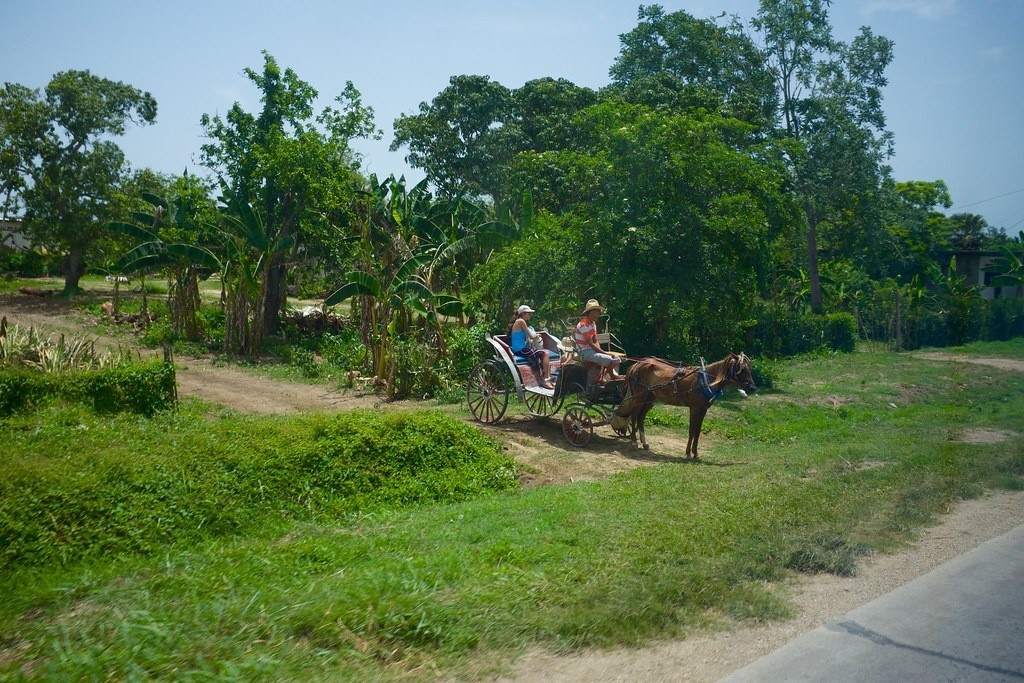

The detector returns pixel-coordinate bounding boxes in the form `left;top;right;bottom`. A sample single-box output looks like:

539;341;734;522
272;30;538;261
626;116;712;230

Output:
536;335;538;338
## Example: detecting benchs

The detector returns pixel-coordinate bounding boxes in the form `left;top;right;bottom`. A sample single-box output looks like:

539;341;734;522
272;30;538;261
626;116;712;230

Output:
563;313;628;394
493;331;561;388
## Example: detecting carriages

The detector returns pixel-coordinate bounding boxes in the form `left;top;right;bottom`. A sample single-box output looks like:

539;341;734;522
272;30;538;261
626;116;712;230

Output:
468;328;757;460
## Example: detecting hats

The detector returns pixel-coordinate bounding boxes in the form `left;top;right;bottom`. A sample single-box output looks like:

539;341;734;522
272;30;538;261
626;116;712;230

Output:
556;337;574;352
582;299;605;314
516;305;535;314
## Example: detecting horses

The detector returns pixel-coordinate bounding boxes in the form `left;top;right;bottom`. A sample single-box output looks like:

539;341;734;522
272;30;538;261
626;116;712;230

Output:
625;351;757;460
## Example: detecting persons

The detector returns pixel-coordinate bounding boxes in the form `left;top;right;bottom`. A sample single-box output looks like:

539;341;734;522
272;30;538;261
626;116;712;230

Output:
507;305;557;389
552;337;574;385
575;299;626;379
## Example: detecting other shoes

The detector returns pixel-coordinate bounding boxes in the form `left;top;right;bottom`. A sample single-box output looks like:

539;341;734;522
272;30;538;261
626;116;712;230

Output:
611;374;624;379
595;379;607;384
542;378;555;389
